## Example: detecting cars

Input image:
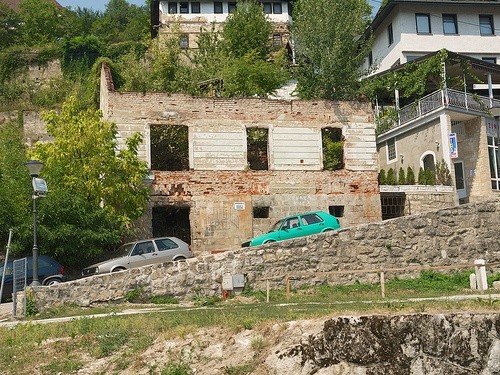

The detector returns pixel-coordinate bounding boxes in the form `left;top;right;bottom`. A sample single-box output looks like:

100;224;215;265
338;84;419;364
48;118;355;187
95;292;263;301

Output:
78;236;193;280
241;208;342;250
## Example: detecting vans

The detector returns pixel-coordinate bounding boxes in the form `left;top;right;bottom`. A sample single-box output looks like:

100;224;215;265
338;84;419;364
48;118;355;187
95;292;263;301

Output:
0;257;67;303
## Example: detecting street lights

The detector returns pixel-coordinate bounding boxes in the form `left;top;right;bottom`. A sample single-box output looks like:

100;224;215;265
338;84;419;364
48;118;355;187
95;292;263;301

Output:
23;159;47;286
